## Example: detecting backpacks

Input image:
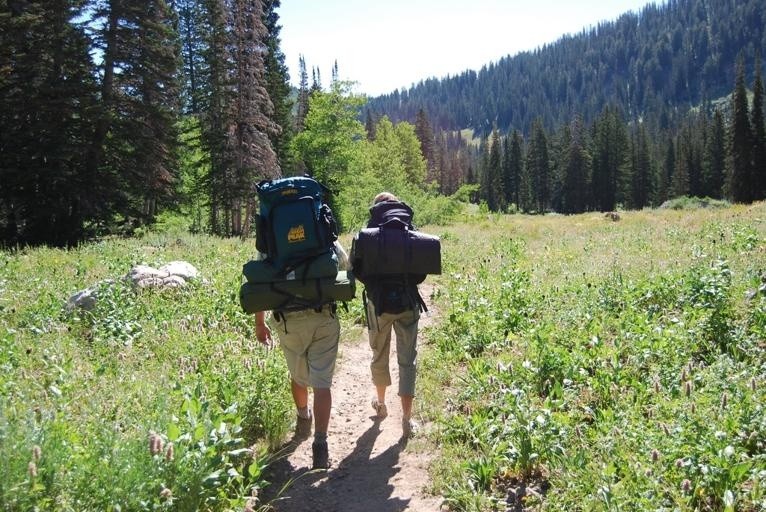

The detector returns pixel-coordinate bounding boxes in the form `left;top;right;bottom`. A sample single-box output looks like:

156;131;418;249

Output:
240;176;354;315
351;200;440;276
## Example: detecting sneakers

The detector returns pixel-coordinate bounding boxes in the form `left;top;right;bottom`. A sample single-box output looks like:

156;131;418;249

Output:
371;398;418;437
296;411;328;468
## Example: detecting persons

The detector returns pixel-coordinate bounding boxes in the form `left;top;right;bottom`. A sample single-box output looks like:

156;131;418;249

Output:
348;189;431;437
248;214;355;469
